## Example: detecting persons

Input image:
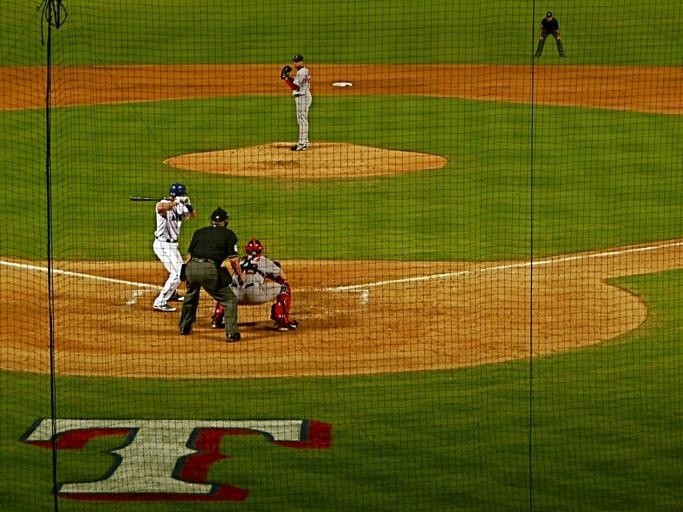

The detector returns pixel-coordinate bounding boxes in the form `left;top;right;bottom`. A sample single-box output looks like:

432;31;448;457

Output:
209;237;299;332
177;206;241;343
279;53;312;153
532;11;566;60
150;182;198;313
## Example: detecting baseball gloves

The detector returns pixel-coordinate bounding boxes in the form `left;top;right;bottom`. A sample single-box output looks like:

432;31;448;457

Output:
280;66;291;80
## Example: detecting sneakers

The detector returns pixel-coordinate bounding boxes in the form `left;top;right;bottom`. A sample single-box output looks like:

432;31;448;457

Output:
278;320;298;331
170;293;184;301
152;304;176;312
179;328;194;335
211;321;226;329
226;332;241;342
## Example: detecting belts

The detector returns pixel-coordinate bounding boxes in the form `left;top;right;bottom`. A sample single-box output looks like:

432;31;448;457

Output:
189;257;214;263
230;283;254;289
155;237;178;243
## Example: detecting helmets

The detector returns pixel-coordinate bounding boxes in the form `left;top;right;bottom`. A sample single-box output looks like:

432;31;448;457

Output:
293;54;303;62
210;207;228;228
244;238;263;256
291;144;306;150
169;183;188;197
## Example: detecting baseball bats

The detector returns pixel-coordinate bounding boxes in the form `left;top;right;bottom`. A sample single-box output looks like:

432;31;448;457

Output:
129;195;188;203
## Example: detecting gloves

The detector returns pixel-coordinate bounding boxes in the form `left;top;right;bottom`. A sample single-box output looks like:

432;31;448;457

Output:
182;195;191;207
174;195;182;205
